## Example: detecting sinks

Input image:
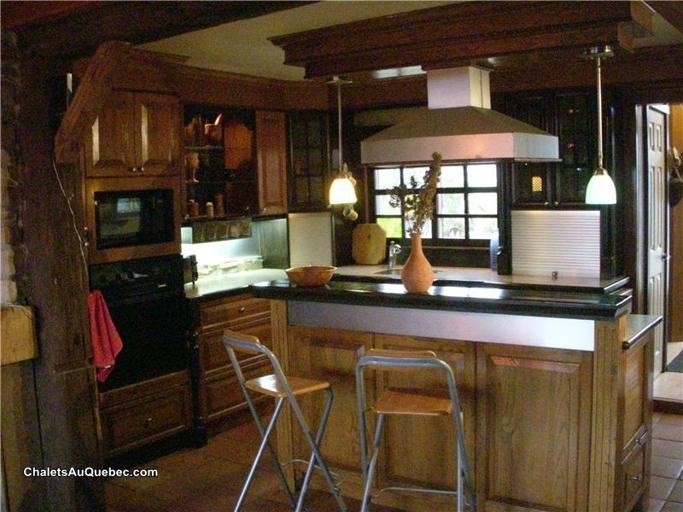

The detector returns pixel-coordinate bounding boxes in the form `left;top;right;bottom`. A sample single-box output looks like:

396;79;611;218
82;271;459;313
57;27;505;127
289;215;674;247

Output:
375;269;442;276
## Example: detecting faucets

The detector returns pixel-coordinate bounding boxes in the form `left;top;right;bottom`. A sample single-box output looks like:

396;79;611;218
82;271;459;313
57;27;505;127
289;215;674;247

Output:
389;241;401;268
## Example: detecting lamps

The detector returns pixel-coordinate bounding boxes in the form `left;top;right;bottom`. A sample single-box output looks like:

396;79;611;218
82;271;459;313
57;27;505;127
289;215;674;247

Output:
327;72;359;205
579;44;619;204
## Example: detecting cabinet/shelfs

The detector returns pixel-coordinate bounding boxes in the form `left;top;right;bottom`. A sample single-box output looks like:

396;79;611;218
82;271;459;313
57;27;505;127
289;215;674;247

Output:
495;90;626;279
70;53;336;453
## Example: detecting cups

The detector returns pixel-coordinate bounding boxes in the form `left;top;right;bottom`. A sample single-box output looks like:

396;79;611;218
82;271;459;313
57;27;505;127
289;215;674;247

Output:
342;204;358;221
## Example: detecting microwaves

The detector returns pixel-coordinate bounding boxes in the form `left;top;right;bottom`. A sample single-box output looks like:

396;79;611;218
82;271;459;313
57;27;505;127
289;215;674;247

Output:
91;187;175;250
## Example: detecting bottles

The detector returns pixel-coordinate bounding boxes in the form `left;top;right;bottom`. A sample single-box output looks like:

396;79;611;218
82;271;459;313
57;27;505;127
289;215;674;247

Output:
206;202;214;220
490;228;504;271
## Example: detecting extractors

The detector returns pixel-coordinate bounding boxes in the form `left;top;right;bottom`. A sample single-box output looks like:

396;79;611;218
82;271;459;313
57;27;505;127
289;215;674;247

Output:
360;65;560;168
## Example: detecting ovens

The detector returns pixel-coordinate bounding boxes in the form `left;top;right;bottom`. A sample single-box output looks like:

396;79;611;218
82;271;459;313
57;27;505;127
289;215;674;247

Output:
87;254;189;395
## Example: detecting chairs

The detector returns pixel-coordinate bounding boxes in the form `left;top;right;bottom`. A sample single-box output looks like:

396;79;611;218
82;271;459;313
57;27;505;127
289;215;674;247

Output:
353;346;476;511
217;328;347;512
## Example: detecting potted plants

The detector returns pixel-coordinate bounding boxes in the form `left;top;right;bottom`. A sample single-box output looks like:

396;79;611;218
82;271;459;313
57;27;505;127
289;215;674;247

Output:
386;149;443;295
